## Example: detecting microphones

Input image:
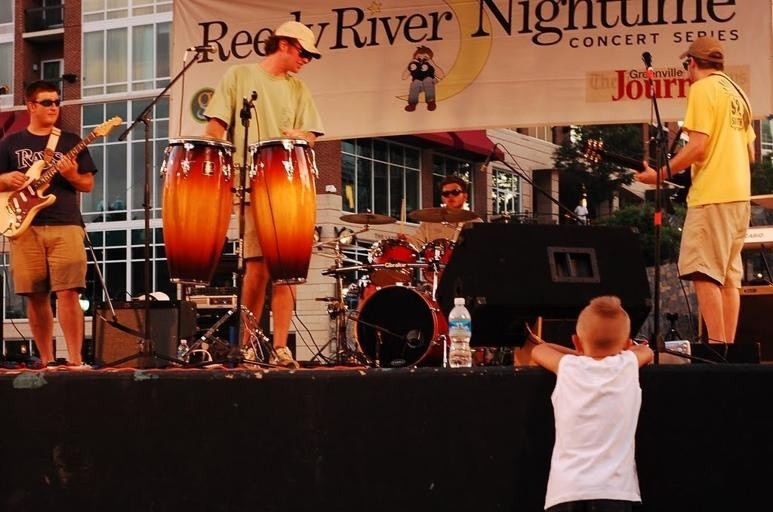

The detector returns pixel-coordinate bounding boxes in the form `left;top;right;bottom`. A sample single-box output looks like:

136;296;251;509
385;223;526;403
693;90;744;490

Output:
641;51;653;85
480;144;497;172
187;44;217;54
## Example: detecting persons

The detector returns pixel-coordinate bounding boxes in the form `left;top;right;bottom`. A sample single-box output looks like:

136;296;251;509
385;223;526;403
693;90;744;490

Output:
203;22;325;366
398;177;484;251
0;81;97;368
531;296;653;511
634;38;757;361
403;47;443;111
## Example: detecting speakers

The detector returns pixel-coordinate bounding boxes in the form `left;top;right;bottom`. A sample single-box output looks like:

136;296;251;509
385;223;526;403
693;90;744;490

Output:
436;223;652;347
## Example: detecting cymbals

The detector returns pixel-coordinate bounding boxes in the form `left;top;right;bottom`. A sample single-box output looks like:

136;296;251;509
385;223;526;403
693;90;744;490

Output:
401;203;478;224
338;208;396;225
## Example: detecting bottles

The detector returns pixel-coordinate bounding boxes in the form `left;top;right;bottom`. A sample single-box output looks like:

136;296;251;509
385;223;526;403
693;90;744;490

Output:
178;339;190;365
448;298;471;368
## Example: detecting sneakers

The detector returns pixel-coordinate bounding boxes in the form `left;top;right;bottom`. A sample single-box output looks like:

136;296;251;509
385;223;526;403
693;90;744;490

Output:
269;345;300;370
240;346;259;368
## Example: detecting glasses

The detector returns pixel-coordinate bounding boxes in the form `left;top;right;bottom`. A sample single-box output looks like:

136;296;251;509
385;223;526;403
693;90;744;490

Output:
441;189;466;198
32;99;61;107
682;57;691;72
288;42;312;59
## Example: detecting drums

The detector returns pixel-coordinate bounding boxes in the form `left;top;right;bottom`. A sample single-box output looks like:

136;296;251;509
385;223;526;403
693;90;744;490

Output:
352;281;451;368
247;136;317;286
161;135;237;287
421;237;455;283
365;237;417;278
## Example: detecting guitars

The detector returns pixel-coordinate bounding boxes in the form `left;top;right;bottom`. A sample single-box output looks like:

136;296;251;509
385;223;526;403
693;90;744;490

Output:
563;130;699;196
0;115;123;240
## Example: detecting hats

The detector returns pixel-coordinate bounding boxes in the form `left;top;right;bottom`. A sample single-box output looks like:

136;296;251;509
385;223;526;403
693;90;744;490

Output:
679;36;725;63
274;21;322;60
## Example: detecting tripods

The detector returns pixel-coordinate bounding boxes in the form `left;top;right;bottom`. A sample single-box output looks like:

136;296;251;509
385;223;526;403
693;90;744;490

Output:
305;227;369;368
183;157;283;363
92;53;201;370
642;79;723;365
181;105;288;368
